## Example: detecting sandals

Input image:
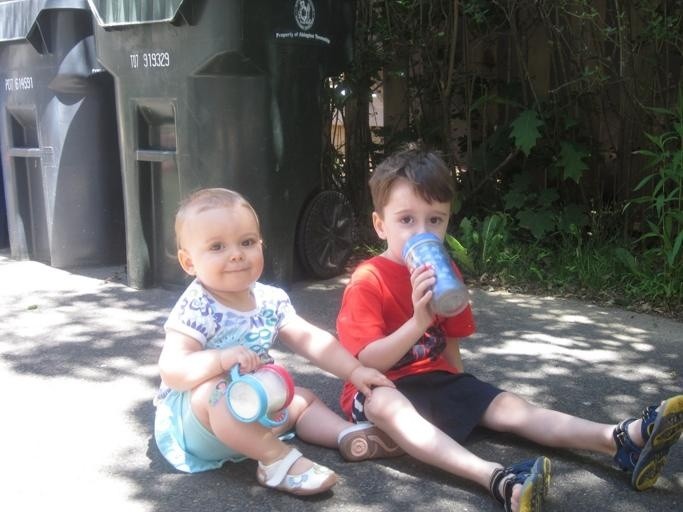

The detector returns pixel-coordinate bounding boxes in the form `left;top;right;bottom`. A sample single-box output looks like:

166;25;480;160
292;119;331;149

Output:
490;454;552;512
612;395;683;492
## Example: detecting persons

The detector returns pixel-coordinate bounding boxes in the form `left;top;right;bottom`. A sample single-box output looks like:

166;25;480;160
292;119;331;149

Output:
152;184;406;499
333;144;683;511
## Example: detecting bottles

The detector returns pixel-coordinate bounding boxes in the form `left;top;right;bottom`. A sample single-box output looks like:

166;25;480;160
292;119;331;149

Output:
401;231;471;319
225;360;293;428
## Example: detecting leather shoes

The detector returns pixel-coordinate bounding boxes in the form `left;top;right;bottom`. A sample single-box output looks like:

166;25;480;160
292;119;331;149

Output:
255;450;338;497
337;422;408;463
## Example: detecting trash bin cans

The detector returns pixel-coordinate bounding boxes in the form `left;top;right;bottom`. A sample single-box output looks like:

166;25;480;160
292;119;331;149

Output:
1;0;126;271
87;0;358;290
